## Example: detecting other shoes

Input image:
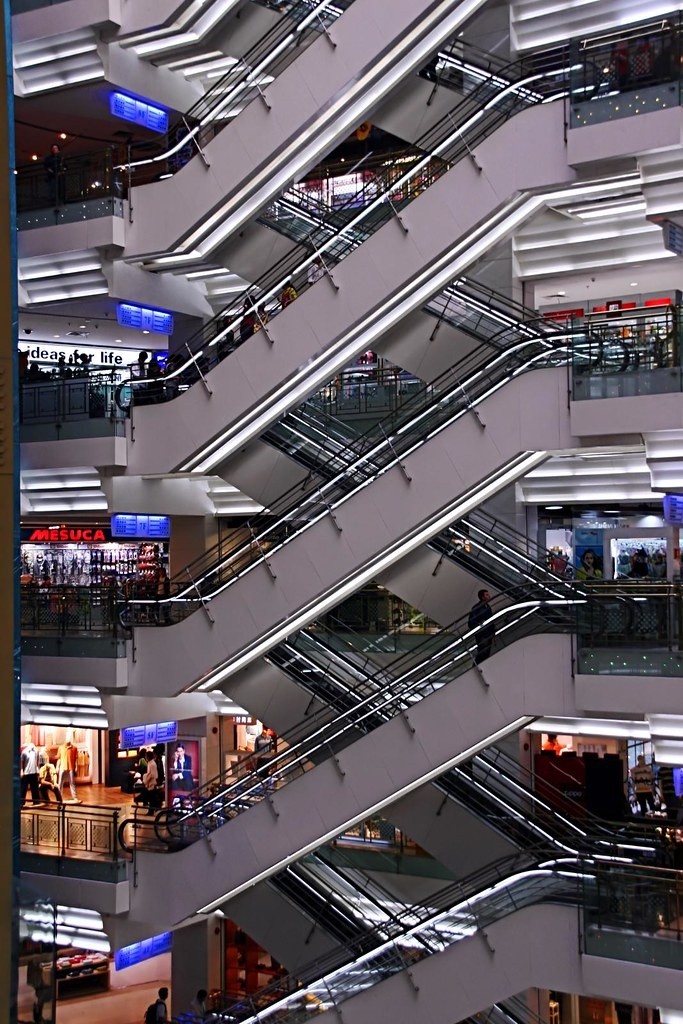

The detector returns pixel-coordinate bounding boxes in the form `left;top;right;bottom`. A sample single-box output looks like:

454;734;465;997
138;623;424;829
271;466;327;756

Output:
134;797;138;805
146;811;154;816
144;803;148;806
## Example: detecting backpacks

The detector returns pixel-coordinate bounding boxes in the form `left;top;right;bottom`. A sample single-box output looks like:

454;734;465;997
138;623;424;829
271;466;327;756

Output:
144;999;167;1024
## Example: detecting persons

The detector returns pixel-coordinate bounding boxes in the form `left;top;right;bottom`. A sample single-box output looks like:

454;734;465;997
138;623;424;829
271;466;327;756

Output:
171;744;193;791
631;755;683;820
56;742;79;802
39;763;62;806
20;743;40;807
143;987;168;1024
419;55;439;82
468;589;496;668
277;280;298;311
147;294;270;405
44;144;69;206
152;568;170;626
190;989;207;1023
576;549;603;579
136;743;165;816
551;550;569;575
255;724;272;778
308;257;331;287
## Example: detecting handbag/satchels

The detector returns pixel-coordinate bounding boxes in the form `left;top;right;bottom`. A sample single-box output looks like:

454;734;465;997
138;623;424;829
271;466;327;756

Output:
97;965;107;971
137;759;147;773
58;972;67;979
42;954;98;971
82;968;93;974
68;970;80;977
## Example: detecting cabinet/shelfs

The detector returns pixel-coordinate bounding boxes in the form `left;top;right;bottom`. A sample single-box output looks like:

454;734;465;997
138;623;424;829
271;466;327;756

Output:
219;918;289;1007
28;952;110;999
23;543;170;625
553;312;672;369
